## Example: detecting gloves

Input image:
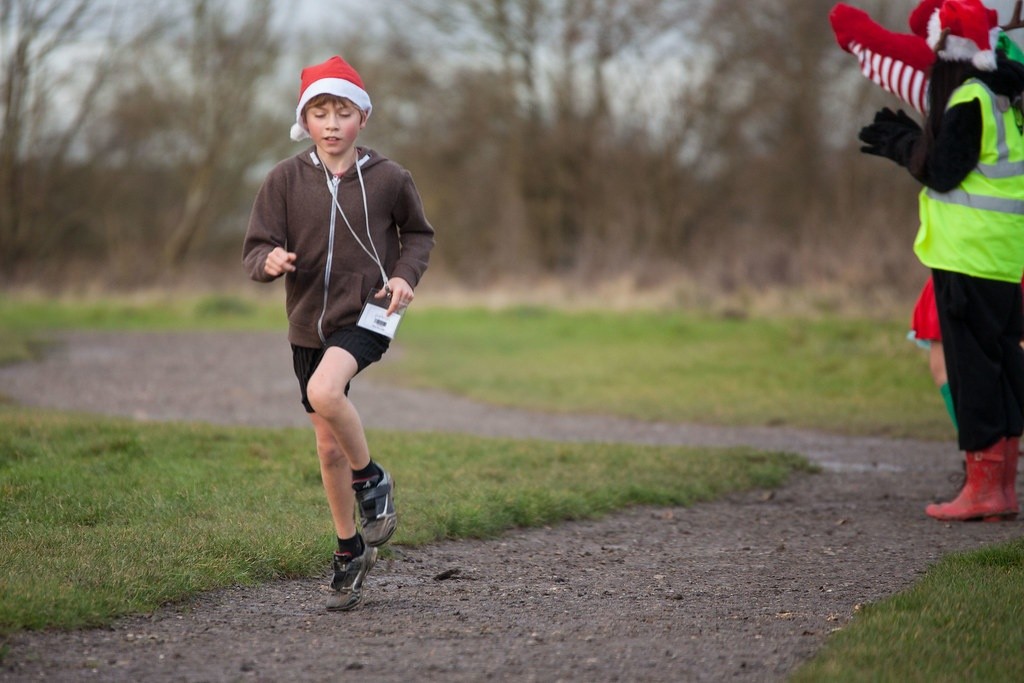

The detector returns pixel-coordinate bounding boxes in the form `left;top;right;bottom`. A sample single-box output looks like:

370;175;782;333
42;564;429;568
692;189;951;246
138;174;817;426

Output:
859;107;923;164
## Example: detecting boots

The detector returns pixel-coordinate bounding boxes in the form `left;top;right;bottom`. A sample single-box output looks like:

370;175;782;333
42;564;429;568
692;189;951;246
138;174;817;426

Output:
1006;437;1019;519
924;438;1007;521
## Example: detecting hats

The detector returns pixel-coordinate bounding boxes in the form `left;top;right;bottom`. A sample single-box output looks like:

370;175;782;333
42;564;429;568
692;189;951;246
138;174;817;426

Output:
289;56;372;142
926;2;1000;71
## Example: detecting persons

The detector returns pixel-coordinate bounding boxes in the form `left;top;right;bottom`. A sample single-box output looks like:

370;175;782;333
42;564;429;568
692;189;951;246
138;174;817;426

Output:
240;55;435;610
824;0;1024;521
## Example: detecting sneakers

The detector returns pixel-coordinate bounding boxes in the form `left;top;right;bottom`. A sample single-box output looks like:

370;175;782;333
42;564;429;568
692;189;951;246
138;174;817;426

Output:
326;545;378;610
355;462;398;546
